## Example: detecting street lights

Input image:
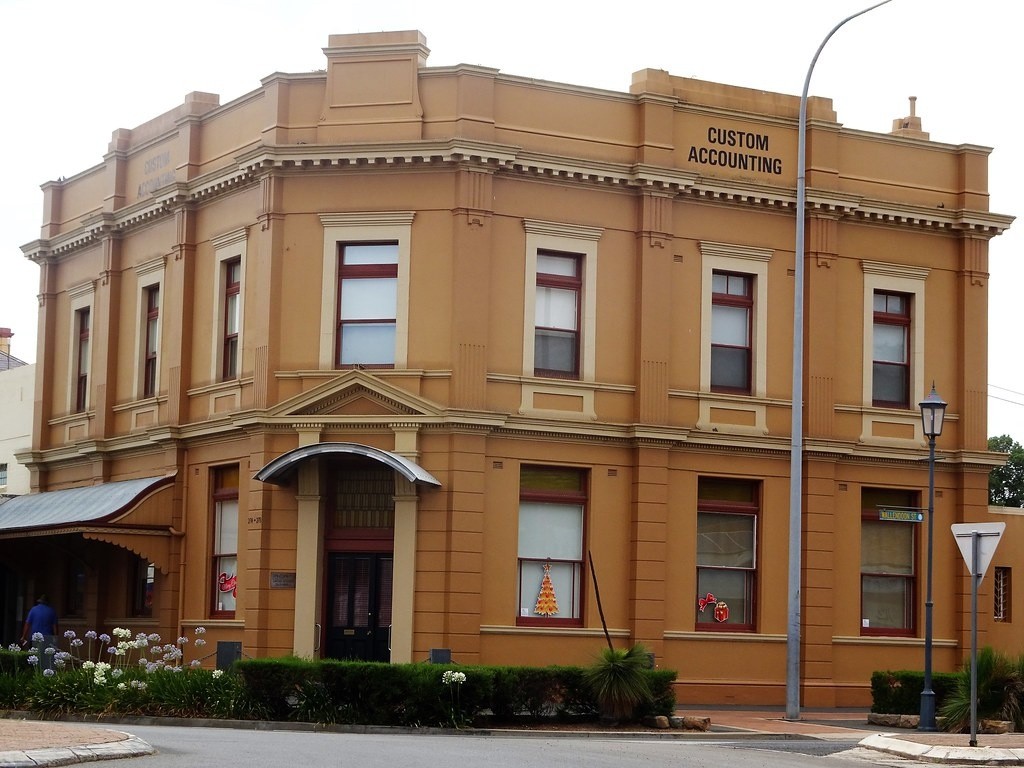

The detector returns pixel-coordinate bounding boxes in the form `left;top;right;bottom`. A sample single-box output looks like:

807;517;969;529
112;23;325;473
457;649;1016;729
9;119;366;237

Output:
918;380;949;734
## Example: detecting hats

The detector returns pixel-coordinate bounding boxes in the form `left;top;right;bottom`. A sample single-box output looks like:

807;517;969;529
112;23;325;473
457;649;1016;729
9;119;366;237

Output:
37;594;49;604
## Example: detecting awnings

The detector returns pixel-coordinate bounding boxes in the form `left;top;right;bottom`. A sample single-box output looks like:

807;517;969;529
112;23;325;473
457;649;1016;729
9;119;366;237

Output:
252;441;442;493
0;473;177;577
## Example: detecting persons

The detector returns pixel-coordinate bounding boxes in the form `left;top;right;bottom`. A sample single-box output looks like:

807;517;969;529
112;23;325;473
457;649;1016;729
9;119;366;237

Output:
20;593;59;671
